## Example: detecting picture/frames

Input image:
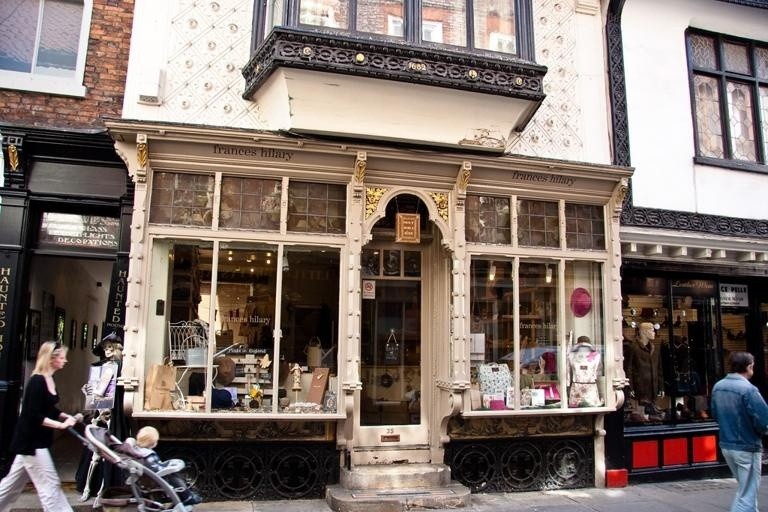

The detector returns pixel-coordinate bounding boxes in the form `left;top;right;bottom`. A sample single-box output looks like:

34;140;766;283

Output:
25;306;98;363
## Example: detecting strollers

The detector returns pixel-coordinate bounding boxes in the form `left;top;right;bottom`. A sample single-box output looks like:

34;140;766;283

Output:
65;413;200;512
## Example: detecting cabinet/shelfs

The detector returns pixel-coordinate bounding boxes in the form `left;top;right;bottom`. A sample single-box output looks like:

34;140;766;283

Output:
471;281;556;368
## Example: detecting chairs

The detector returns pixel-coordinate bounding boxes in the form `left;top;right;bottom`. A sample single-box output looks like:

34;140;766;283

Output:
163;318;220;411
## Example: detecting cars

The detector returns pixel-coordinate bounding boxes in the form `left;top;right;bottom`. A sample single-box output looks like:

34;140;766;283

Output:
492;345;557;375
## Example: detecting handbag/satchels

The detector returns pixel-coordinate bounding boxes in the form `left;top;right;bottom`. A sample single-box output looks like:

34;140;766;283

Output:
143;356;176;410
476;362;510;393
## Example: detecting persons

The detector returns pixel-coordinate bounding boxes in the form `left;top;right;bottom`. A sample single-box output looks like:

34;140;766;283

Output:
79;333;122;509
132;424;204;505
0;340;83;511
626;322;666;418
709;350;768;511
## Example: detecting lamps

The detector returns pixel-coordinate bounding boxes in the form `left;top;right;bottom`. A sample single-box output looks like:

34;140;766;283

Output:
488;260;498;281
545;263;553;284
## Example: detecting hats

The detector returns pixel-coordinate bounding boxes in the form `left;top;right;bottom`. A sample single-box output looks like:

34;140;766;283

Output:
571;336;595;352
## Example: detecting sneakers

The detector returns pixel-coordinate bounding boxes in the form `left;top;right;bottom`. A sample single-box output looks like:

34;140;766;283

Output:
183;495;201;506
